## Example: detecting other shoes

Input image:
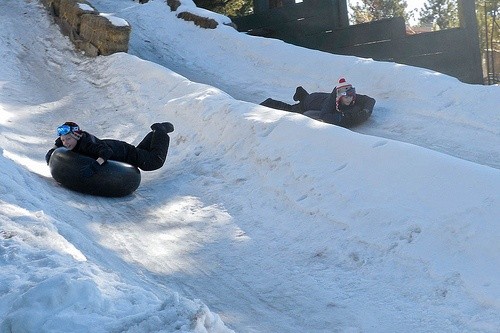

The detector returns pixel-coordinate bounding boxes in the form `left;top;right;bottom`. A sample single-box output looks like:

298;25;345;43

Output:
293;86;304;101
150;123;166;134
258;98;272;106
161;122;174;133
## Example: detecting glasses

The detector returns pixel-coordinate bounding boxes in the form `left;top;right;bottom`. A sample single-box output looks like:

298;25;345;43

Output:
342;88;355;96
56;124;80;136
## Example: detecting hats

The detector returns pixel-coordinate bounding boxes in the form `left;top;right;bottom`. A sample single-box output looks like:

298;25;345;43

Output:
62;122;81;140
334;78;355;116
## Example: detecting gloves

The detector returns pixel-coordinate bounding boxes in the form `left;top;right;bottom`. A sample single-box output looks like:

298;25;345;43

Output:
80;161;100;176
46;149;55;165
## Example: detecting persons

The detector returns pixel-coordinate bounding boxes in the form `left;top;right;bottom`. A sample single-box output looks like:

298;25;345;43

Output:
45;122;174;171
259;78;376;127
